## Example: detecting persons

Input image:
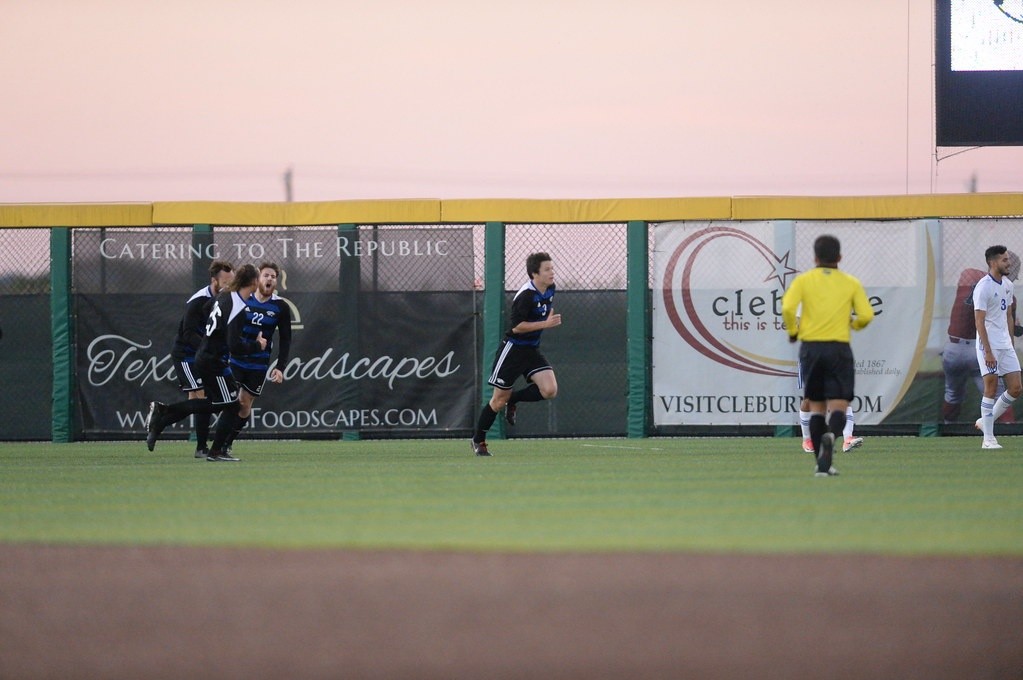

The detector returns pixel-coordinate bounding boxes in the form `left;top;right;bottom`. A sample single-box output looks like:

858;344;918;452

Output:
942;251;1023;423
147;261;292;461
471;252;562;456
783;234;874;477
972;245;1023;448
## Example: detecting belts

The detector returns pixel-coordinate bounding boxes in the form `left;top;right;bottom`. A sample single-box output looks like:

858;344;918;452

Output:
949;336;971;344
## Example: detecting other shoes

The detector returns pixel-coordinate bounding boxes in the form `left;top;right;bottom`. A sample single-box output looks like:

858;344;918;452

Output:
814;432;840;476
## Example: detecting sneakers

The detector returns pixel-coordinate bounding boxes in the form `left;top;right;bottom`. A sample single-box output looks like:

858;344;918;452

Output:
207;451;241;462
226;445;233;457
843;436;864;452
975;418;998;443
505;401;517;426
470;436;494;457
981;440;1003;449
802;438;815;453
146;432;161;451
195;447;210;458
146;400;168;432
